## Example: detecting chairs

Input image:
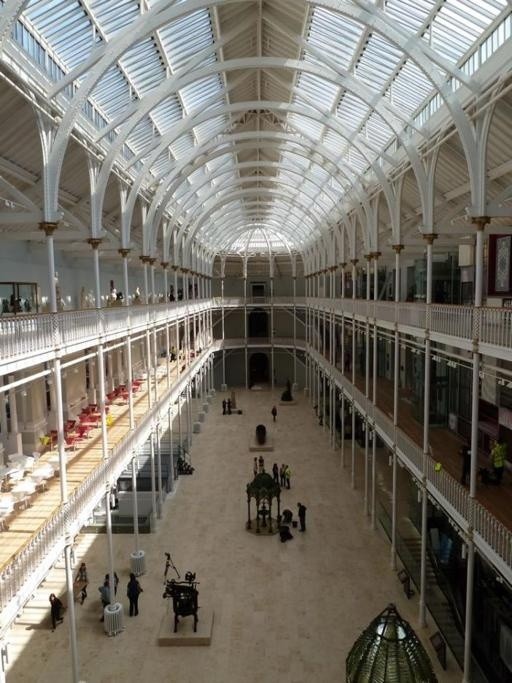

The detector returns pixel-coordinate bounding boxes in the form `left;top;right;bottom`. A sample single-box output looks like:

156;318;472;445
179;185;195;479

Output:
0;339;196;537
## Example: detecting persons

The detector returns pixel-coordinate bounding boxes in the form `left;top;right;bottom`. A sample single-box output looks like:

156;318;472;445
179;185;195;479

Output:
113;570;118;596
286;379;291;390
458;439;471;489
223;397;226;414
280;463;285;486
98;580;110;621
345;349;351;372
127;572;139;616
49;593;63;632
169;345;176;361
284;464;291;488
259;455;264;473
297;501;307;531
488;439;508;479
74;562;88;605
253;456;258;478
273;463;279;483
272;405;278;420
227;397;232;413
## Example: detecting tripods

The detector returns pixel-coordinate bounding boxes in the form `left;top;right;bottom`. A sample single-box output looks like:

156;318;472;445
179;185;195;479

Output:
164;552;180;585
